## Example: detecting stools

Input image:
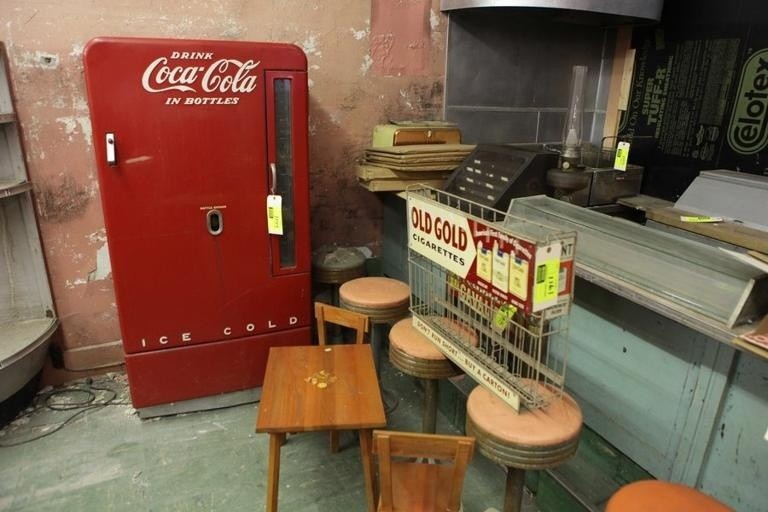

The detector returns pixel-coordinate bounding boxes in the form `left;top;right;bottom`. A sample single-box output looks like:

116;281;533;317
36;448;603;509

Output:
339;277;412;411
606;481;734;512
389;316;477;434
312;235;377;312
465;379;583;512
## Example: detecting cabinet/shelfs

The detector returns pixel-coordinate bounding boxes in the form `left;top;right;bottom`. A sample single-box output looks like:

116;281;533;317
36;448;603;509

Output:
0;41;63;424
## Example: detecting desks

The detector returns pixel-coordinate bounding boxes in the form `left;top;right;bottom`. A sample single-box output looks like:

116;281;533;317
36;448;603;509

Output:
255;343;387;512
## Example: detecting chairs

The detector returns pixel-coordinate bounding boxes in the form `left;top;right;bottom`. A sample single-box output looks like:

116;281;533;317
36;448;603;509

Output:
283;302;371;454
367;429;476;512
